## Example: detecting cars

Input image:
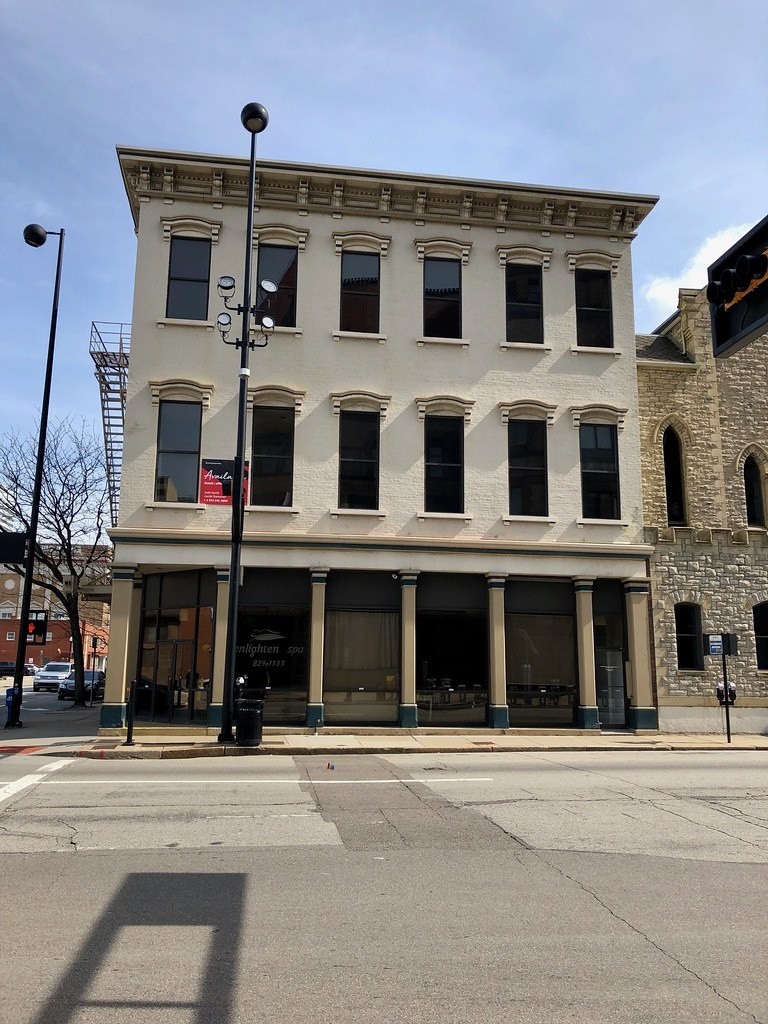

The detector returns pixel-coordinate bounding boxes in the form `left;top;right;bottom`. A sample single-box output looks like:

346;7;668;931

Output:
23;663;41;676
0;661;29;678
58;669;106;701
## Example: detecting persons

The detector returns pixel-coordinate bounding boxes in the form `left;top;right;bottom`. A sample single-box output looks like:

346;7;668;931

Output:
233;675;249;699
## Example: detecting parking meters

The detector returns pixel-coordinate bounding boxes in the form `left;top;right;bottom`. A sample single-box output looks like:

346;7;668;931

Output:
235;676;244;699
715;680;737;743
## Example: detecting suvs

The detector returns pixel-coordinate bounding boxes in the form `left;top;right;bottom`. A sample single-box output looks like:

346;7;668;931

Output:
32;660;75;692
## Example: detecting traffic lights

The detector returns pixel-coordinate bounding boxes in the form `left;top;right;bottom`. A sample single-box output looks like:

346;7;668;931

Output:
28;622;37;635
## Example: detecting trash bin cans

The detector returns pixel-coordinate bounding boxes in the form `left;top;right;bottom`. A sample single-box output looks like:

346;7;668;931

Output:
233;688;269;746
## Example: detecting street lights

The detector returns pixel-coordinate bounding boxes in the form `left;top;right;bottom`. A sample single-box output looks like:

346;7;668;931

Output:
1;223;67;730
216;102;279;744
